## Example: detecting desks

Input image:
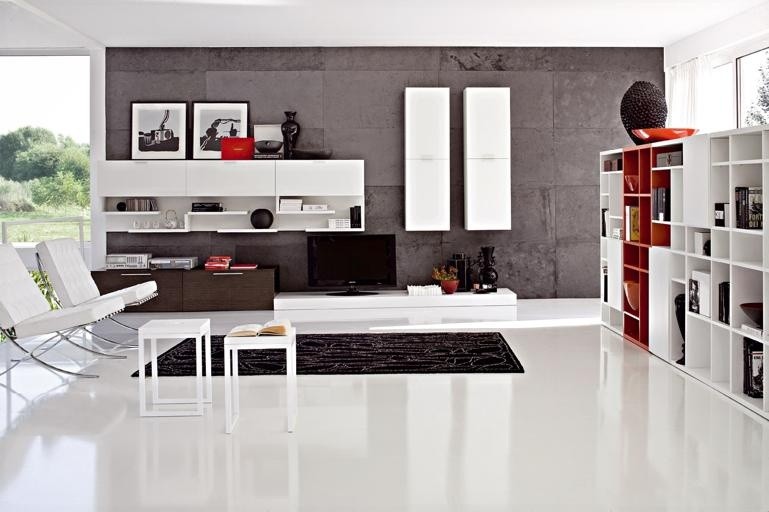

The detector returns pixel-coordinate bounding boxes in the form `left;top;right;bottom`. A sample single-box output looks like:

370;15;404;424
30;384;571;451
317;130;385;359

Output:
184;267;281;308
91;268;183;311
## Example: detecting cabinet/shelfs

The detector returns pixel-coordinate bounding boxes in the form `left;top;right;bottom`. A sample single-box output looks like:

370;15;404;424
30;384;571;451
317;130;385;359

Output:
599;123;768;423
96;158;364;235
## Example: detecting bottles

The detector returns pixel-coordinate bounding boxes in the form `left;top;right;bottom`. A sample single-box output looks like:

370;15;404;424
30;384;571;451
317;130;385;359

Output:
715;203;725;226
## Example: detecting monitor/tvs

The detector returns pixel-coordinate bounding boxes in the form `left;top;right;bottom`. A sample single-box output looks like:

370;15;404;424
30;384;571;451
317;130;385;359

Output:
307;234;397;296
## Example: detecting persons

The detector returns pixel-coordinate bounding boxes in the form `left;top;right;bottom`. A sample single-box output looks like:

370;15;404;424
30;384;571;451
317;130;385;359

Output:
664;153;672;166
689;281;700;313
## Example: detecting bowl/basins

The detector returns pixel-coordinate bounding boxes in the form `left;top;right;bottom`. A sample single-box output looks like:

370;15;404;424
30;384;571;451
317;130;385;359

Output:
293;148;332;159
623;280;639;315
625;175;639;193
740;302;763;328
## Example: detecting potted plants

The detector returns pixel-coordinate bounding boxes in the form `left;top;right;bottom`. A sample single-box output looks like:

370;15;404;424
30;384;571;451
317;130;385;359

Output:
431;265;459;293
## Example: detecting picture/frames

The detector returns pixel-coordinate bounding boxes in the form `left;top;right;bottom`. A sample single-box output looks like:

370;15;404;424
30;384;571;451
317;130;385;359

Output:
191;100;251;159
129;100;188;160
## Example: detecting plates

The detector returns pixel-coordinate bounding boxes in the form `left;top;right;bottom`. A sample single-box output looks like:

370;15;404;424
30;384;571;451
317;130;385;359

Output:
631;128;695;142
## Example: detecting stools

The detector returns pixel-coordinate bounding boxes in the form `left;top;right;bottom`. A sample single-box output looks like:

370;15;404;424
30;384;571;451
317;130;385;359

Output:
137;319;213;418
224;327;297;433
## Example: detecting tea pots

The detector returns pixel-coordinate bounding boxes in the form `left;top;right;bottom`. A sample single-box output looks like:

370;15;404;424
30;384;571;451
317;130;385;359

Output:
164;209;178;228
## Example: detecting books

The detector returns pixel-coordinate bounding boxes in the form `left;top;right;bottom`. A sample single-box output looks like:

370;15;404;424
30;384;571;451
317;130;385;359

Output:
228;321;291;336
601;208;609;237
192;206;222;212
653;187;671;221
601;268;608;302
192;202;221;208
626;205;639;243
743;337;763;399
279;198;361;229
719;281;730;325
735;186;762;229
203;256;258;270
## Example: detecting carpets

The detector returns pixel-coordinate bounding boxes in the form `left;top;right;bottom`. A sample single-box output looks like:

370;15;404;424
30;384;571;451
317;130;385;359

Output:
131;331;525;387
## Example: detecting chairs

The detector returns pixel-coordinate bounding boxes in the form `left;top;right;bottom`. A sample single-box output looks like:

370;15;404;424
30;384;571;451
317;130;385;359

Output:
35;238;159;347
0;241;128;378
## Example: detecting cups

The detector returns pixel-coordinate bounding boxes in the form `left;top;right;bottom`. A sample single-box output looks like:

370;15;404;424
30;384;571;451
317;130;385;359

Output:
180;221;184;228
132;218;159;229
